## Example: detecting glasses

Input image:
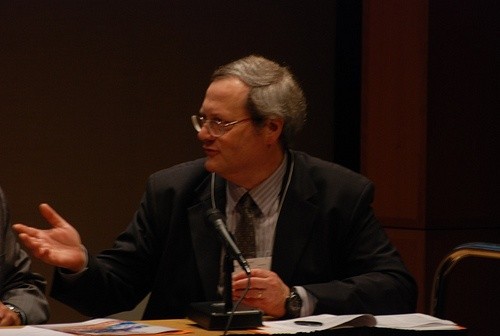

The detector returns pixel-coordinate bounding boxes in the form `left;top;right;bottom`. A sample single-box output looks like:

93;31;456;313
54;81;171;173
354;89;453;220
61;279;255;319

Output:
191;115;257;137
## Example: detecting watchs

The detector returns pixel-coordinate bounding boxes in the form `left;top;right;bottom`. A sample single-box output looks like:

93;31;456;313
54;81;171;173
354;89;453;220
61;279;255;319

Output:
278;286;303;320
4;304;27;325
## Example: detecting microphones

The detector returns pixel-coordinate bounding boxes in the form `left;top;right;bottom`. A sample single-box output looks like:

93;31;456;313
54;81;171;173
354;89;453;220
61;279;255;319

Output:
204;208;251;274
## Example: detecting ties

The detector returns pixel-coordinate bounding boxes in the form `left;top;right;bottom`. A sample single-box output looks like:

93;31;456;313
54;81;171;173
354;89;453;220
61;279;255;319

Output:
224;192;263;312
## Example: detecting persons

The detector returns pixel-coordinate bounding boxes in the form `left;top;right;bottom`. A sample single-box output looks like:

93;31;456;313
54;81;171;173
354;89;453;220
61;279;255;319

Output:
11;55;418;320
0;184;52;326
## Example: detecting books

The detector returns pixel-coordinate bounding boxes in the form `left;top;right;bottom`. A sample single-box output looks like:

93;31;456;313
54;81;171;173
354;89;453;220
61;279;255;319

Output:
258;311;466;335
0;317;193;336
